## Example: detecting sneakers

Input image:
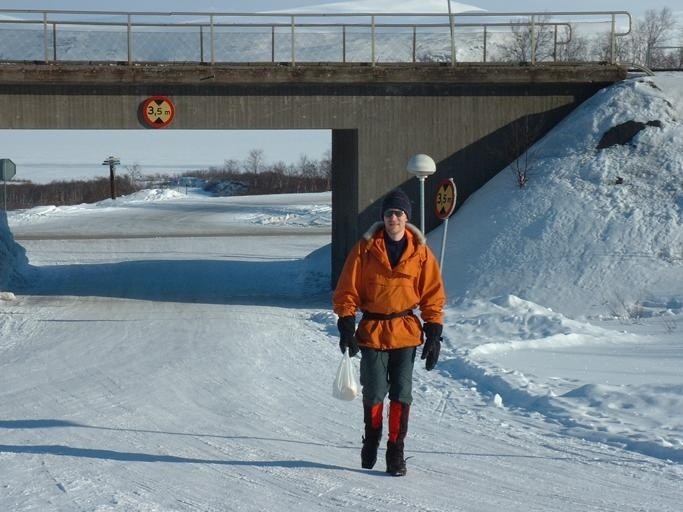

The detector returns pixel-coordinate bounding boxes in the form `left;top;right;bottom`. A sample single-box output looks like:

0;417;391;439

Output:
385;439;407;475
361;425;383;470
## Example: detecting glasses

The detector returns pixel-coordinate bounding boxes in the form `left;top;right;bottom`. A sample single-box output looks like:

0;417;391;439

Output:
383;210;403;217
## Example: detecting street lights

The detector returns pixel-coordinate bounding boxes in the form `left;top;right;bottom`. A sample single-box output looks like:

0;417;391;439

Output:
406;153;437;235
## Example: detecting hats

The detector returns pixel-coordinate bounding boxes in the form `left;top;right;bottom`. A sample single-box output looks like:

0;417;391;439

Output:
381;186;412;222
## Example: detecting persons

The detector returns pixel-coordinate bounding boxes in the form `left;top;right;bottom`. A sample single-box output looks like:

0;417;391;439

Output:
331;188;447;478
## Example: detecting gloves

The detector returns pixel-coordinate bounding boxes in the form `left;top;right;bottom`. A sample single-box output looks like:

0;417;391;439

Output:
421;320;443;371
336;315;362;357
